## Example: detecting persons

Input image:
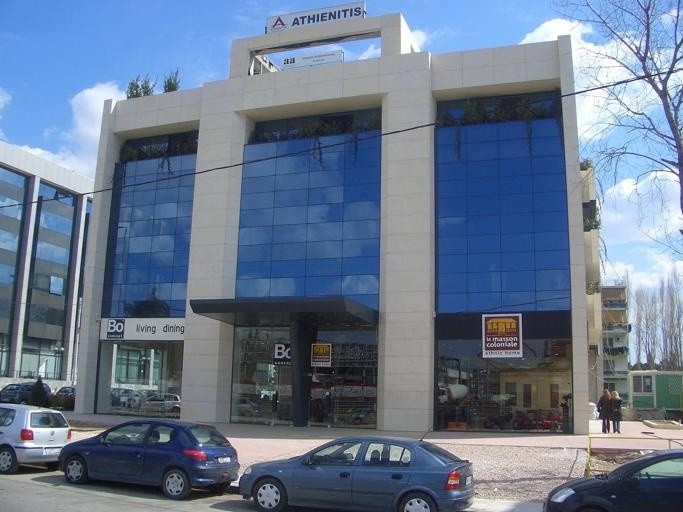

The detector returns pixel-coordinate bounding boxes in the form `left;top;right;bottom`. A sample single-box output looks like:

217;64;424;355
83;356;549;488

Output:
597;389;611;433
609;391;623;432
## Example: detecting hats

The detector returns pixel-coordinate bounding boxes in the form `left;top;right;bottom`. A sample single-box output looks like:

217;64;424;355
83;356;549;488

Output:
0;382;75;410
110;387;179;413
237;435;474;512
231;396;266;417
542;447;682;512
59;419;240;500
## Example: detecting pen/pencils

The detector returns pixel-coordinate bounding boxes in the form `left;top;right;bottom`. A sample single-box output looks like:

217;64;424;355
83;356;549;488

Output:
0;402;76;473
